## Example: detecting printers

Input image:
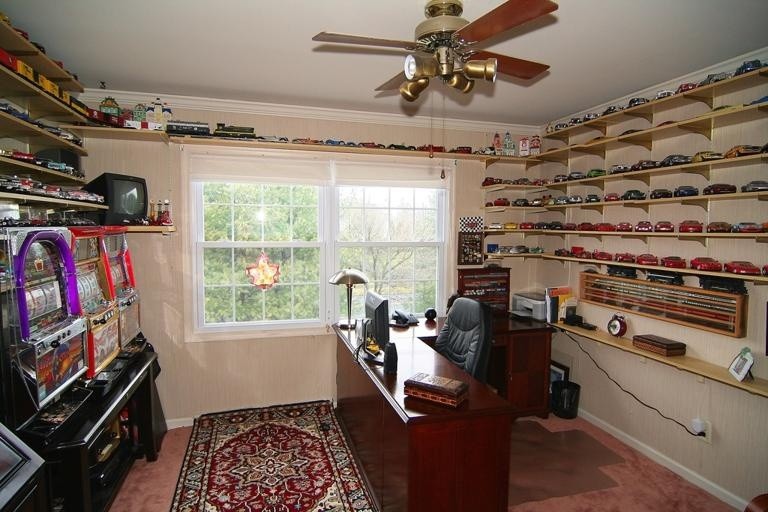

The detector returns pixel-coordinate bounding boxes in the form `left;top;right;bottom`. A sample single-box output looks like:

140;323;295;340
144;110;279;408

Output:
507;290;548;323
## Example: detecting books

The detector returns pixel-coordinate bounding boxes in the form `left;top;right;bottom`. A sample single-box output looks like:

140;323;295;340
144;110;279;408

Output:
404;371;468;408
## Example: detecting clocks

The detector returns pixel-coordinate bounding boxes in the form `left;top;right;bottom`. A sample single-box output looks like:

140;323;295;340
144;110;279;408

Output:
607;312;628;339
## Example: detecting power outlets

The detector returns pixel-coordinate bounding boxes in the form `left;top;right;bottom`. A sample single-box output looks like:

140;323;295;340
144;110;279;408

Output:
696;420;712;444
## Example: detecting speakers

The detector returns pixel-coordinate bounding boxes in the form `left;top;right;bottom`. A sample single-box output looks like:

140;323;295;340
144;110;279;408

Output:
384;341;398;375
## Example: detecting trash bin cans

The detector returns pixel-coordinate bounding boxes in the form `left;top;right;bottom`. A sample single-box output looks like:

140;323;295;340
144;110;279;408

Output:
552;380;580;419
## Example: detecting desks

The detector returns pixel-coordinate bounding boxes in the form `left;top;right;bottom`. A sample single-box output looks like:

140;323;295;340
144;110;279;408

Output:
331;312;553;511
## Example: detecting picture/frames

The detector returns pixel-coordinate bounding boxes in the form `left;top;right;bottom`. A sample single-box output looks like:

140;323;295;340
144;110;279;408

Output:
549;360;569;397
728;347;754;382
457;231;484;265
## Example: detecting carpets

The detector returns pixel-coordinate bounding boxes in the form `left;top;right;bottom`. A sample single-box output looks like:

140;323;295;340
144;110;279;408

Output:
170;399;379;512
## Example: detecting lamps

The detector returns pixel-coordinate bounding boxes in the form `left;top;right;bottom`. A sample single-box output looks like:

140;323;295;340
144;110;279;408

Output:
328;268;369;329
397;54;497;102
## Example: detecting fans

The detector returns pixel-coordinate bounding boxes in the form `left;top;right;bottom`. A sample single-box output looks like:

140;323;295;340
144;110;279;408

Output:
312;0;558;91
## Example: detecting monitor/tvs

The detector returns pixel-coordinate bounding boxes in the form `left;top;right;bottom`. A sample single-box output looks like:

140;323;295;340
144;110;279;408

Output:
81;172;149;227
357;289;390;365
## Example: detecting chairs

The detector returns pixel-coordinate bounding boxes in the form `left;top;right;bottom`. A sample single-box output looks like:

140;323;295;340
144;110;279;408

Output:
433;297;491;383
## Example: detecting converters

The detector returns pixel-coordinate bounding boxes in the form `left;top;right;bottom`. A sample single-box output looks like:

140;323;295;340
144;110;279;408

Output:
691;419;703;440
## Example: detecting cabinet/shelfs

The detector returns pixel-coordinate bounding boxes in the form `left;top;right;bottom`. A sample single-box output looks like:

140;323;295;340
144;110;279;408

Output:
1;9;108;235
457;267;512;317
0;420;47;512
59;122;176;235
15;332;169;512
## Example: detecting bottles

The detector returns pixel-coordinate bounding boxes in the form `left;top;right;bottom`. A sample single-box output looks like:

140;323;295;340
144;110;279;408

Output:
494;129;515;156
519;135;530;157
150;199;173;226
530;134;541;157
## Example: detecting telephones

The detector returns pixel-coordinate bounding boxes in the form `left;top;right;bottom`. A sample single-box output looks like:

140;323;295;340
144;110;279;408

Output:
392;310;419;325
